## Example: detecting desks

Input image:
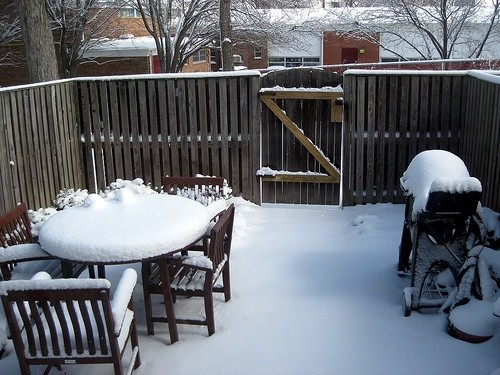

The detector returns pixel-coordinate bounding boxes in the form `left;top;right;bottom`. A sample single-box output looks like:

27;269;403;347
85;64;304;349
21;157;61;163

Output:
39;194;209;344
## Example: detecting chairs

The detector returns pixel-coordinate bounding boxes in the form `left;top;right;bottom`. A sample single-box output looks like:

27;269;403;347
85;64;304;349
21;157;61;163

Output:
0;202;142;375
142;177;235;336
